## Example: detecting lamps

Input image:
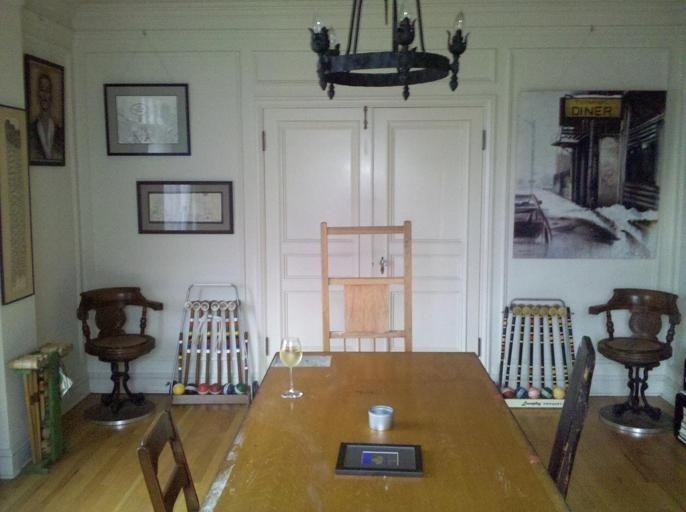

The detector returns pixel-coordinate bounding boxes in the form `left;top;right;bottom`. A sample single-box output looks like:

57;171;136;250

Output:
307;0;470;100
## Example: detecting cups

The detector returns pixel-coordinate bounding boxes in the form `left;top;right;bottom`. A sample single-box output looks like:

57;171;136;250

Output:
368;404;394;432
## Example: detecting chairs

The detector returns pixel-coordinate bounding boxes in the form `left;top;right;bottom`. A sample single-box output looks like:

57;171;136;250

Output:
76;286;163;426
546;336;595;501
137;410;200;512
589;288;681;433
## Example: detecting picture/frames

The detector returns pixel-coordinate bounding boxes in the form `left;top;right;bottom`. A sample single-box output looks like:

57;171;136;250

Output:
334;443;423;478
0;103;34;305
103;83;191;156
136;182;232;233
23;53;65;166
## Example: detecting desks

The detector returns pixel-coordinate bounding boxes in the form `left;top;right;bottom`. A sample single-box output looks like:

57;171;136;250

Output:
200;352;569;512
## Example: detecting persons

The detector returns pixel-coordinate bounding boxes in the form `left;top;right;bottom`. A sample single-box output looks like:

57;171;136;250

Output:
29;75;64;162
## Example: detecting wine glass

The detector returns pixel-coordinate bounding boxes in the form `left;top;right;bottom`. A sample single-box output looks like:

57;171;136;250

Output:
277;335;304;398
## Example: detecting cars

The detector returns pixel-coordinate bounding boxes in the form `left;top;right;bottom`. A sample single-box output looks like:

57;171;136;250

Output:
513;193;555;259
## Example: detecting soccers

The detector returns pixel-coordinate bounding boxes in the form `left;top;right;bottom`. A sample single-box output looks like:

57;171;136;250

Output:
501;386;565;399
173;383;245;395
